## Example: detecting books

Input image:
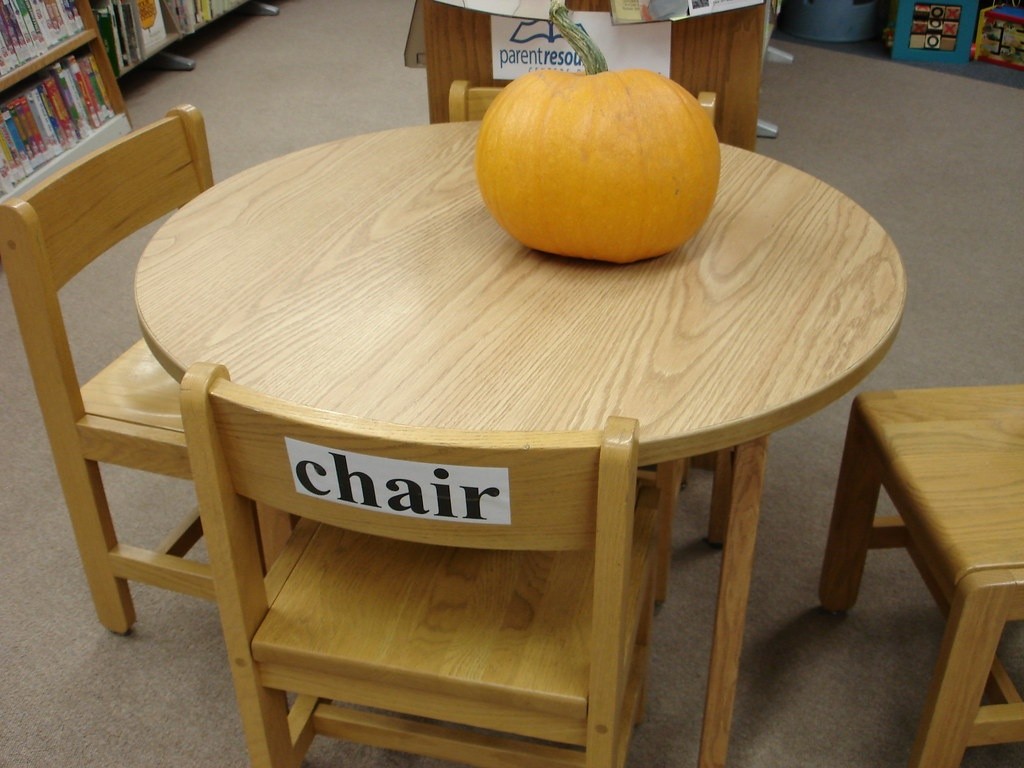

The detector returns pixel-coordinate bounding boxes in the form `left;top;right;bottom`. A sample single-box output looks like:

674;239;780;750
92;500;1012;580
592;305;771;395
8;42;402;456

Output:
0;0;248;199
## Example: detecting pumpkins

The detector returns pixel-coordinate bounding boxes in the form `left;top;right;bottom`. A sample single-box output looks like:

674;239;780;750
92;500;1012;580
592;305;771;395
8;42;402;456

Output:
477;1;719;264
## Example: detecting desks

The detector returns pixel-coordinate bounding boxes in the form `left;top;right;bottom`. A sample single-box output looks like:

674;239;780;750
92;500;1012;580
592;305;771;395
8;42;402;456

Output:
133;120;907;768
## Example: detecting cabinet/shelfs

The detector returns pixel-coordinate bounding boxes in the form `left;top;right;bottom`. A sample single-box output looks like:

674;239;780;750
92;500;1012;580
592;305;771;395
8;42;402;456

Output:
1;0;134;206
421;0;770;153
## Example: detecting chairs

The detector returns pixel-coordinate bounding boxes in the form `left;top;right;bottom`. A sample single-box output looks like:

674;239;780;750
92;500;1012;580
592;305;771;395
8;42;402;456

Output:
1;102;300;635
176;361;670;768
816;383;1024;768
446;81;718;128
